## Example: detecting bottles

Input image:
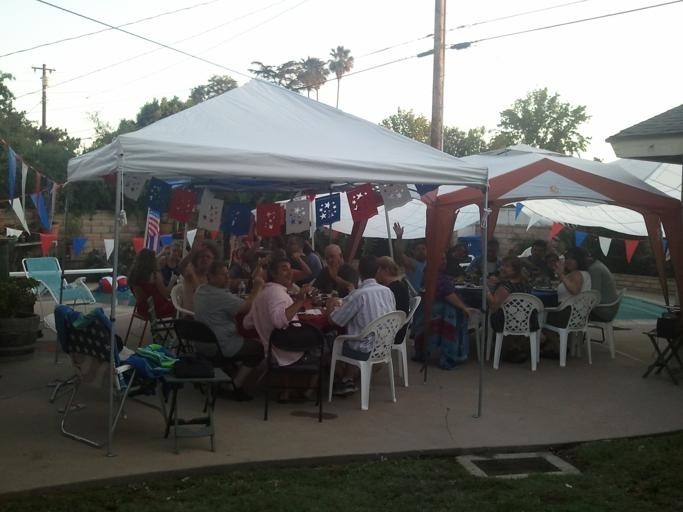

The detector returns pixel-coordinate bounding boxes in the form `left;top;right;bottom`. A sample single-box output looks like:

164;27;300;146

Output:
331;287;339;306
312;280;323;307
238;281;246;296
552;274;560;289
470;269;481;286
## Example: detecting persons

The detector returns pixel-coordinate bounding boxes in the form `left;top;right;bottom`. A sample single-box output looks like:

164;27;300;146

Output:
540;248;591;348
160;233;324;292
176;239;223;324
130;252;179;321
325;257;396;396
243;259;331;402
375;255;408;344
393;235;567;294
415;253;470;365
482;255;540;355
311;246;357;299
193;262;263;402
578;244;620;322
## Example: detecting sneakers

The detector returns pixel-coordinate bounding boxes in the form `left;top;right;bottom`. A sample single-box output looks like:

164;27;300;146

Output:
332;378;358;397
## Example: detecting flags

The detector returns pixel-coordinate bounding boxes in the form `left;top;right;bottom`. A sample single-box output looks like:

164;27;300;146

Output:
146;208;161;251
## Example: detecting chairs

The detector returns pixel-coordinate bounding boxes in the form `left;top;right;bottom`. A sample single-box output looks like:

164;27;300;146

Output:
262;321;324;422
487;293;544;372
328;310;407;414
125;282;183;352
391;295;421;387
171;284;197;352
22;258;96;328
463;307;486;364
579;288;625;359
175;320;241;411
543;289;601;367
50;304;146;447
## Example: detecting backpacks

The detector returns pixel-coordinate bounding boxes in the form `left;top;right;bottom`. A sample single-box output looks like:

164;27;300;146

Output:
169;352;216;380
127;344;177;383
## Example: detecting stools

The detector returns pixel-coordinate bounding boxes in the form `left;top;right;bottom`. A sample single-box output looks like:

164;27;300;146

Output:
640;327;683;386
159;369;231;453
